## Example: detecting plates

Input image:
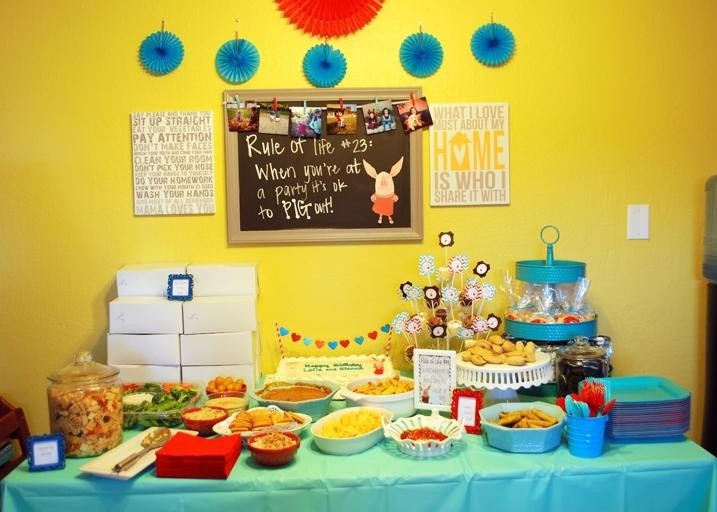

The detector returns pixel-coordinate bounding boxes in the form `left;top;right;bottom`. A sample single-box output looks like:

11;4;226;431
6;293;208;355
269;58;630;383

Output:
577;375;692;442
79;425;199;482
212;409;313;446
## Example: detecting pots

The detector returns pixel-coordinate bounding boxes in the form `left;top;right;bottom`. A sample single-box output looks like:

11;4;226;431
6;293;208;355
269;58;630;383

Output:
340;375;418;421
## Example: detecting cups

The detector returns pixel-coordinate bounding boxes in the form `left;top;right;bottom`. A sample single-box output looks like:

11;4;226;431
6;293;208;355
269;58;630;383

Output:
565;413;609;459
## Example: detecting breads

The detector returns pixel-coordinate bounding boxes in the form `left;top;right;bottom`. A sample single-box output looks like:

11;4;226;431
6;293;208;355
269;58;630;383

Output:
505;302;597;324
461;334;536;367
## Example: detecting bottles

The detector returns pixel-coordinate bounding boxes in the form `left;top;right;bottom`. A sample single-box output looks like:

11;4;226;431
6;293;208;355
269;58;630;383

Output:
47;350;124;458
555;335;612;399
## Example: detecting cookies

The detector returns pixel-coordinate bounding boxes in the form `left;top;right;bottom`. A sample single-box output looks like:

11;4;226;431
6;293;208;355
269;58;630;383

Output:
491;406;557;429
353;373;414;396
228;408;305;432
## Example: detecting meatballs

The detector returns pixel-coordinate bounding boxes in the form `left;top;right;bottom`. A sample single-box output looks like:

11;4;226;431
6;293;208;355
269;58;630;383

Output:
207;375;245;393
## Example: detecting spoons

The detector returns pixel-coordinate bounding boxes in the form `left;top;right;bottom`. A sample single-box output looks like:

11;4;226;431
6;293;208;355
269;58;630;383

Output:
557;380;616;417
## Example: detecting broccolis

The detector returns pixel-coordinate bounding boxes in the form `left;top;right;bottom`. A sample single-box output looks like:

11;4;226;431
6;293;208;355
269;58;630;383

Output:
123;380;191;428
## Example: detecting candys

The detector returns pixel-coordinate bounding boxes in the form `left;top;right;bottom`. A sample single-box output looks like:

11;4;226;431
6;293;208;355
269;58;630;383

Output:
394;231;503;363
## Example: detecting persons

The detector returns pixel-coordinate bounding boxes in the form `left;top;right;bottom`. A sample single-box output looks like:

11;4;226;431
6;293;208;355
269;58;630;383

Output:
406;106;423;131
236;106;258;132
334;110;347;131
291;108;321;138
367;107;394;133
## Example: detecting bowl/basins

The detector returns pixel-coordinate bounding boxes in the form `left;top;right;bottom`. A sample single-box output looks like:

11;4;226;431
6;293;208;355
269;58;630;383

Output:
250;377;342;423
478;401;565;454
181;380;250;435
247;432;301;467
311;406;394;456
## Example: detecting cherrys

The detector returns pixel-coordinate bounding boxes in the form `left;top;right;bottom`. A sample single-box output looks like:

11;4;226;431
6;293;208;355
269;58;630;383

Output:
401;425;449;453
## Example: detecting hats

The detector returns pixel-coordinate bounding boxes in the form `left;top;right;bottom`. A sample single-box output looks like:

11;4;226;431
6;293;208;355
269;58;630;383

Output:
314;109;320;113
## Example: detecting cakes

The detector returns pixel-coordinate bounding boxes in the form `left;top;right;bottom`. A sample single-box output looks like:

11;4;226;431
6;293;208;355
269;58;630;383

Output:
275;353;394;383
